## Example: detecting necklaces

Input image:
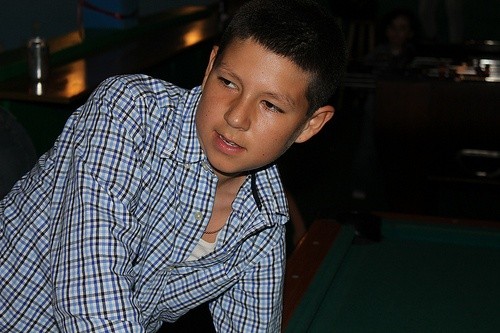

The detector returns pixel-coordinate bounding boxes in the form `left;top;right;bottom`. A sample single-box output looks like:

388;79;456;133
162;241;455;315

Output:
202;226;225;234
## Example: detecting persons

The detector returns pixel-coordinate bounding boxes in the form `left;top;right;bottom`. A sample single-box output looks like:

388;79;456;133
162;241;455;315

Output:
0;0;338;333
381;9;416;71
